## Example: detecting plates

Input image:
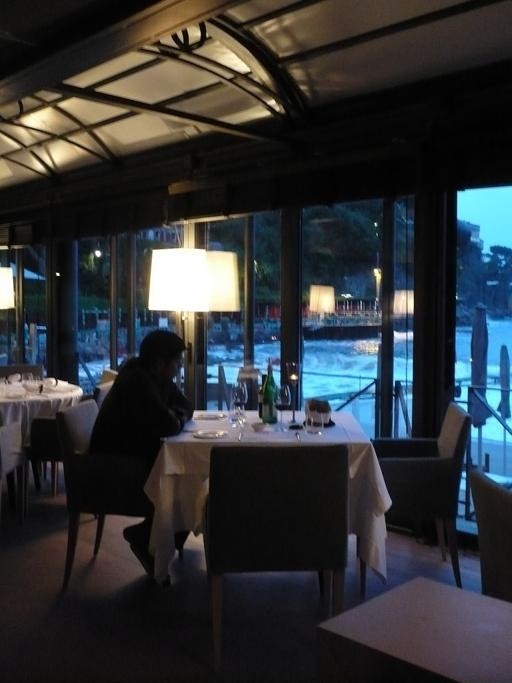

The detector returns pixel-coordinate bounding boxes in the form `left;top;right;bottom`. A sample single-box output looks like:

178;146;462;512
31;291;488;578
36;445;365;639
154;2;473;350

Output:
193;412;229;439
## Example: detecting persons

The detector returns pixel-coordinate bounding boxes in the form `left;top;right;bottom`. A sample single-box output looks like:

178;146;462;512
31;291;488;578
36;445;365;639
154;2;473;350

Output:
72;328;195;587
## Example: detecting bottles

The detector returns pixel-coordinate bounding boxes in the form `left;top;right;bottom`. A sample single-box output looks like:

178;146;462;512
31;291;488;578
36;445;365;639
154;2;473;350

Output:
257;374;269;419
261;364;279;426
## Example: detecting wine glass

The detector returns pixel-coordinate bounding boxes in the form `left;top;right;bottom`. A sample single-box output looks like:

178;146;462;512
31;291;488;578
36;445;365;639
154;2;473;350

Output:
230;382;248;430
275;383;292;432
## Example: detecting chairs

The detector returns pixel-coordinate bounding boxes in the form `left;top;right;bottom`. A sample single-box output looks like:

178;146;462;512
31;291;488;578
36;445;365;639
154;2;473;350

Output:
201;441;350;674
56;398;182;592
84;370;116;399
218;363;234;411
470;469;512;605
94;381;114;408
370;402;473;589
31;399;100;494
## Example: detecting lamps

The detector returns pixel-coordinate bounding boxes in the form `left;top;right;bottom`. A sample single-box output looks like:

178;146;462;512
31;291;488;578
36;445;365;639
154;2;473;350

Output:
392;290;414;316
309;282;336;316
147;245;241;314
0;266;15;311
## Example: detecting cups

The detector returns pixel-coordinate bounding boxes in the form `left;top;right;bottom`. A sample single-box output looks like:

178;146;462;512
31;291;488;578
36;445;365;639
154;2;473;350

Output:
0;372;42;399
306;411;324;434
